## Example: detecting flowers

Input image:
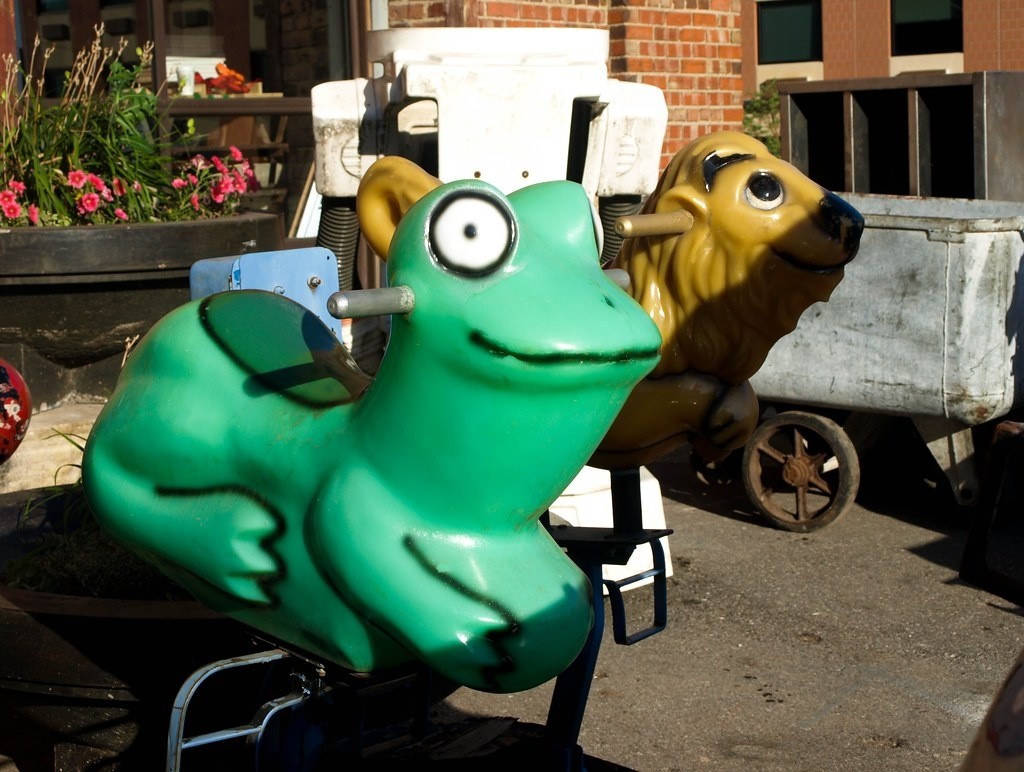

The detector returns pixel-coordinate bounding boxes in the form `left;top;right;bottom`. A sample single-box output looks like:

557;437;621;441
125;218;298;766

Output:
0;21;261;228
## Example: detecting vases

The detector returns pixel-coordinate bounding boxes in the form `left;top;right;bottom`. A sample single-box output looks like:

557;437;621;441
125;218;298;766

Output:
0;207;281;494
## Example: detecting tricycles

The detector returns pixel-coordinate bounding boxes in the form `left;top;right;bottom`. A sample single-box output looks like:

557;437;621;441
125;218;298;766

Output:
728;190;1024;533
